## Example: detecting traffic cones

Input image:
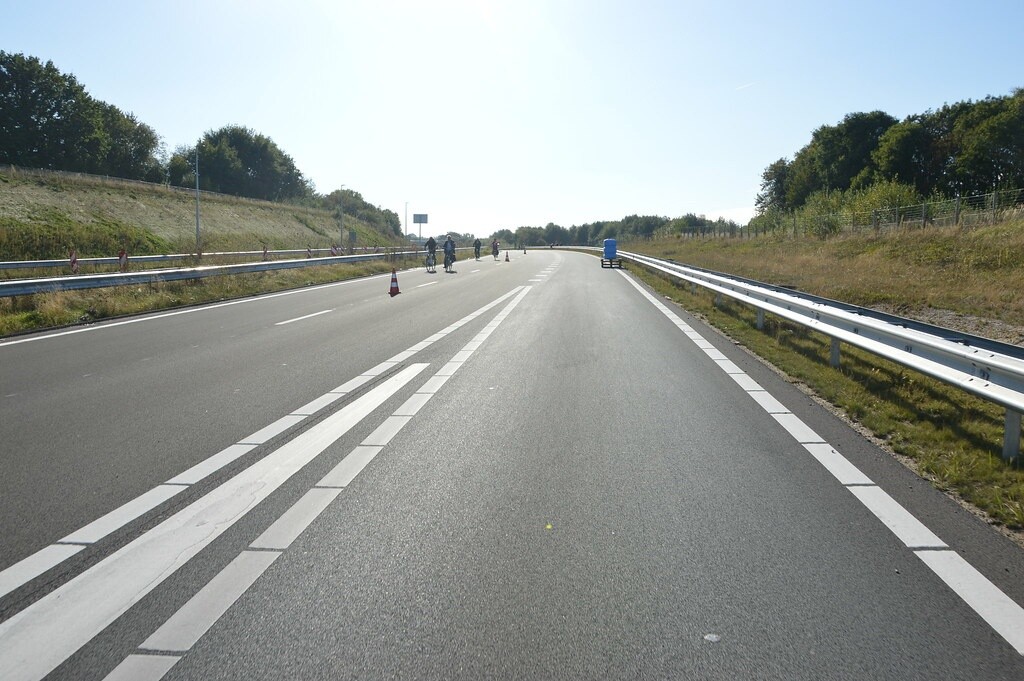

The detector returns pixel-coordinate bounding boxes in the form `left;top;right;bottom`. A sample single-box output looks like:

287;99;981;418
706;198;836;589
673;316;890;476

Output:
387;266;400;294
504;250;509;262
524;247;527;254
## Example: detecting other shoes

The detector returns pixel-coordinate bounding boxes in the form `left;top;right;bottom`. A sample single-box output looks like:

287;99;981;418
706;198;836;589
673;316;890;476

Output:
443;267;446;268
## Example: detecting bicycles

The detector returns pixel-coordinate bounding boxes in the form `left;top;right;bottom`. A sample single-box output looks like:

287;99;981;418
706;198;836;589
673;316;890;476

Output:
474;245;480;259
424;247;437;272
492;245;498;260
442;247;453;273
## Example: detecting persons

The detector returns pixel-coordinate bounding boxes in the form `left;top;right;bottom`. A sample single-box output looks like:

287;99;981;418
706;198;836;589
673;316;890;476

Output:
491;239;500;255
473;238;481;257
442;235;456;267
424;237;438;266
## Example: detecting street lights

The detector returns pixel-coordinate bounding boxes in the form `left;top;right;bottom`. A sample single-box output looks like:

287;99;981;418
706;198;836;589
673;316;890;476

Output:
195;144;206;251
340;183;345;248
